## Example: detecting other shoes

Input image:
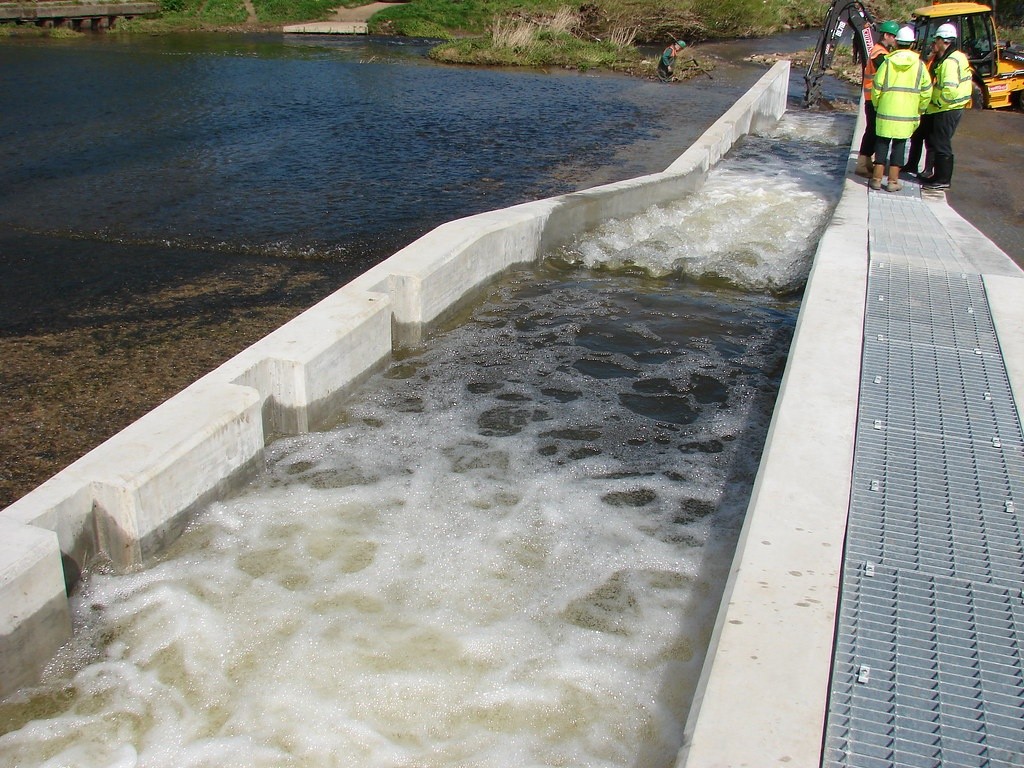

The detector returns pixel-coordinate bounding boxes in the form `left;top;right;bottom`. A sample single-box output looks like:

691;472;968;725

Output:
916;169;934;180
899;161;918;174
921;174;951;191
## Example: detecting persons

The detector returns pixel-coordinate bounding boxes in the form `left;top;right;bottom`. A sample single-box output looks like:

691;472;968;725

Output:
901;23;973;190
855;21;900;178
868;26;933;191
657;41;686;82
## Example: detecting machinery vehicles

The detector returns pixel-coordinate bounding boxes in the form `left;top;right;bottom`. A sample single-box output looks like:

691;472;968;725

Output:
801;0;1023;114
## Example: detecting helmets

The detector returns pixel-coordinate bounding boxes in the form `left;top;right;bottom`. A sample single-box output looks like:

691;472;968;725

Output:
677;40;686;49
894;26;916;42
933;23;958;39
878;20;900;36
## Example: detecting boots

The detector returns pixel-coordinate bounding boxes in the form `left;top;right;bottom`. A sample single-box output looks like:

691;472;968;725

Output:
868;163;902;192
854;154;875;178
